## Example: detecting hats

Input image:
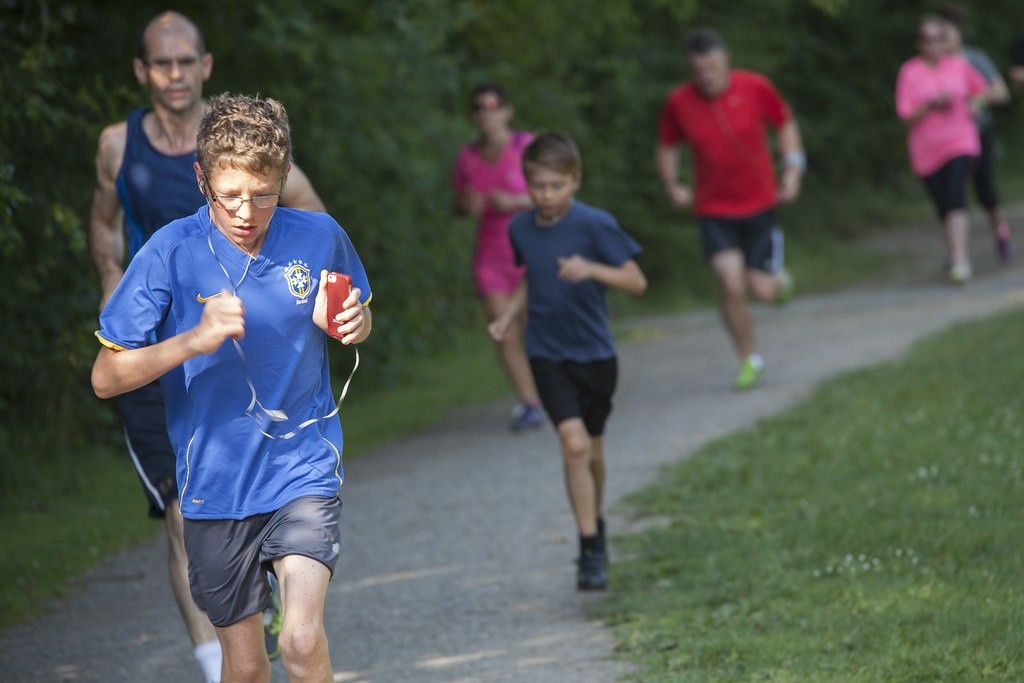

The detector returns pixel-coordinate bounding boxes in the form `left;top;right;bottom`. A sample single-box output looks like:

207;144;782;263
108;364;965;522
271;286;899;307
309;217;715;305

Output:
467;83;507;112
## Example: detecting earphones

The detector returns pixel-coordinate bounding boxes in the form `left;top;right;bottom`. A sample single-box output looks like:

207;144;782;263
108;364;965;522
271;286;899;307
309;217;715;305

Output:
199;179;207;197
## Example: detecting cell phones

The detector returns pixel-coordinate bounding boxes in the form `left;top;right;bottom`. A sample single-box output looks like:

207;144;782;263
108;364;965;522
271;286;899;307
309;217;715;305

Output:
327;271;351;338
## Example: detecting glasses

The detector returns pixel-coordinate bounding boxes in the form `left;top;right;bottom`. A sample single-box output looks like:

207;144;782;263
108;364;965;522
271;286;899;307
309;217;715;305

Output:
202;164;284;210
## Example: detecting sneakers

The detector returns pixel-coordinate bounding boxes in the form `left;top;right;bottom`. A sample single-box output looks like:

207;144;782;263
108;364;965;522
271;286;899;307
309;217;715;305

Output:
941;253;972;284
508;401;543;433
777;268;794;309
261;571;286;660
992;222;1012;258
576;548;608;596
732;354;766;389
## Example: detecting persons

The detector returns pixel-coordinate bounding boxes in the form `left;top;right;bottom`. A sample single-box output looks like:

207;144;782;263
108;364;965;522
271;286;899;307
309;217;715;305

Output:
89;88;378;683
489;131;652;594
446;79;553;433
648;30;803;399
892;11;1023;287
86;9;339;682
